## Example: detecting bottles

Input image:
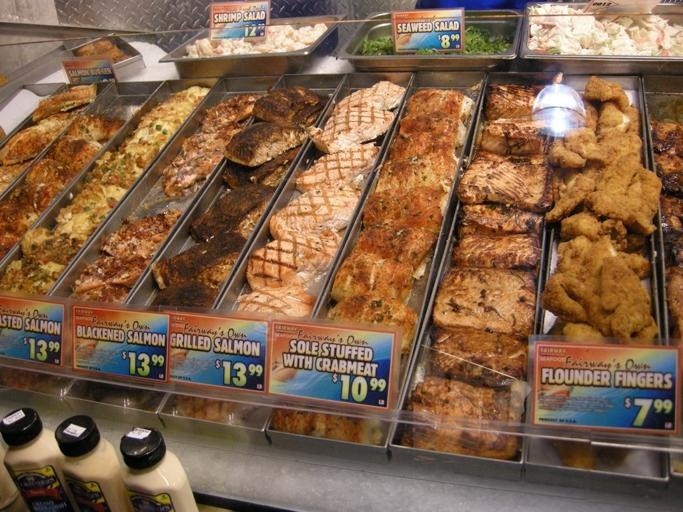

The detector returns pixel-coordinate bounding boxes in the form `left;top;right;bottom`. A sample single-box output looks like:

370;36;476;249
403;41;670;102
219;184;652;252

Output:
120;428;200;511
55;414;132;511
0;407;80;511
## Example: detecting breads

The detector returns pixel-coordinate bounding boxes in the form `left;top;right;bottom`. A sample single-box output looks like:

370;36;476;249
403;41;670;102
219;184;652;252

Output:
328;89;476;355
273;404;382;445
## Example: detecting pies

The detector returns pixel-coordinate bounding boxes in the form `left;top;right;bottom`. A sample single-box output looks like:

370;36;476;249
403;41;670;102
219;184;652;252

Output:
0;85;213;296
